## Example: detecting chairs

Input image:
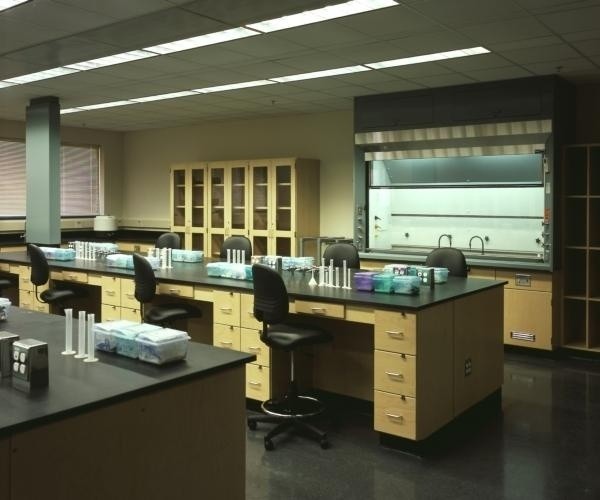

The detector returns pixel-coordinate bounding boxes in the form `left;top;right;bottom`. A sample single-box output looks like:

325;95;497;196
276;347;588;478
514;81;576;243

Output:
220;236;251;260
247;265;330;450
0;278;18;306
133;254;201;329
322;244;360;269
156;232;181;250
427;247;467;278
27;243;89;314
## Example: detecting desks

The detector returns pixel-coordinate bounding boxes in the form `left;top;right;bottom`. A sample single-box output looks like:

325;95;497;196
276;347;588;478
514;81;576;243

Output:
1;307;256;500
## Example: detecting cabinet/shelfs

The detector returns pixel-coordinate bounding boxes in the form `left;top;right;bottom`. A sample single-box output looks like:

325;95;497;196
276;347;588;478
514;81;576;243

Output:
154;282;270;402
0;261;100;322
558;142;600;354
169;161;207;257
100;271;141;323
286;284;504;441
207;161;248;257
466;266;564;352
249;158;319;258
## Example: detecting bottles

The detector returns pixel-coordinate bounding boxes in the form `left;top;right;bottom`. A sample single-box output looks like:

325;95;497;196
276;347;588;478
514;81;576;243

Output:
147;248;172;269
86;314;95;359
78;310;86;356
225;249;245;265
74;241;95;259
65;308;74;352
309;258;351;289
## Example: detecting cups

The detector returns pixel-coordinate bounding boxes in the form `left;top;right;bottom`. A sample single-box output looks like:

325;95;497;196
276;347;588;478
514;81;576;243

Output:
0;298;12;321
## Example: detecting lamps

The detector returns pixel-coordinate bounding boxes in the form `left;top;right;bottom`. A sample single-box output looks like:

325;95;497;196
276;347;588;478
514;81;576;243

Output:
93;216;118;242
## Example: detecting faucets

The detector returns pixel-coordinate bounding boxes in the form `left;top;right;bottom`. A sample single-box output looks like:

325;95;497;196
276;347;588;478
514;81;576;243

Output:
438;233;452;248
469;236;484;255
